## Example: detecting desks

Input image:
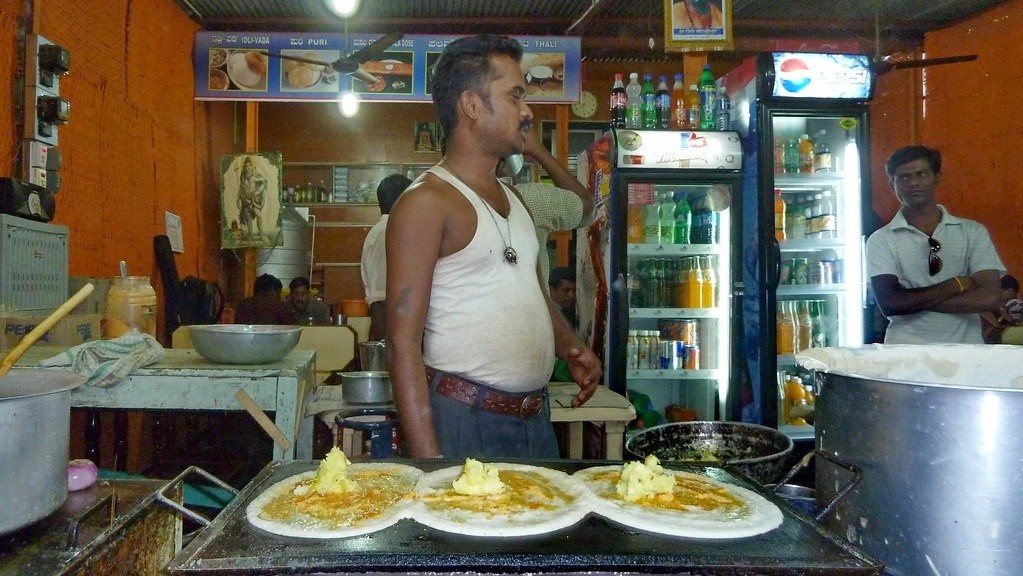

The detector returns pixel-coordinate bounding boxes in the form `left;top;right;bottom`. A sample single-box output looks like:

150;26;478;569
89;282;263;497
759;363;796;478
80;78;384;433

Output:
30;344;321;520
301;375;638;466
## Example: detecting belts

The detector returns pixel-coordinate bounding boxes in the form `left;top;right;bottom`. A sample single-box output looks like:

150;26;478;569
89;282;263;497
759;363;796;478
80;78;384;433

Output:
424;366;547;420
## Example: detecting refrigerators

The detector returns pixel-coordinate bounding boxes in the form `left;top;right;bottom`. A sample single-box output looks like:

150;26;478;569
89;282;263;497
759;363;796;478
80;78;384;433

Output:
714;52;876;451
568;131;745;459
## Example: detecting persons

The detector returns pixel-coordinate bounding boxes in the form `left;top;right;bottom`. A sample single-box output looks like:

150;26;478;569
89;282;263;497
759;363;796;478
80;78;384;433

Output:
864;145;1009;345
439;121;597;306
284;276;333;328
865;210;1023;344
234;272;295;327
549;264;577;338
384;32;603;460
360;173;414;346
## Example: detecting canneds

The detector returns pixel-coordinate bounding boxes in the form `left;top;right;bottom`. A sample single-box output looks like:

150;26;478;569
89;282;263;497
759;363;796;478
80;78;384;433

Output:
656;317;700;370
777;255;841;287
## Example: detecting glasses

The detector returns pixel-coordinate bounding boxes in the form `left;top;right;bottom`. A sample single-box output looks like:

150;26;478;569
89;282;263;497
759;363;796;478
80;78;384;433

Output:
927;236;943;276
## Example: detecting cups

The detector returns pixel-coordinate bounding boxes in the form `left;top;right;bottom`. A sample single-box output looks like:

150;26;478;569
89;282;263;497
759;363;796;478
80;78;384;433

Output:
332;313;347;326
358;341;387;372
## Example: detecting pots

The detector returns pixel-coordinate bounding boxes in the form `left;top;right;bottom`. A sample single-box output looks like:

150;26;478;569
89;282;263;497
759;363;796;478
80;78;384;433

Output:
796;341;1022;574
335;369;393;406
0;364;89;537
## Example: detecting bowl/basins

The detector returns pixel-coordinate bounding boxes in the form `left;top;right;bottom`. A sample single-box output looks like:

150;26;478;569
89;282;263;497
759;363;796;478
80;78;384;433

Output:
210;69;228;90
531;65;553;84
185;323;303;364
209;49;227;68
624;420;793;485
340;299;369;316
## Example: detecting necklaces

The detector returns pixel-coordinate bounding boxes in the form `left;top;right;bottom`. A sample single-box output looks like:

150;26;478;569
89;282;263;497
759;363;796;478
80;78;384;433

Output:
440;156;518;265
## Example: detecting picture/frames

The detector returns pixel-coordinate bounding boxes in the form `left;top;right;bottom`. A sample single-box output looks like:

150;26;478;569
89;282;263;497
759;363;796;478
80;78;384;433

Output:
218;147;284;251
663;0;734;54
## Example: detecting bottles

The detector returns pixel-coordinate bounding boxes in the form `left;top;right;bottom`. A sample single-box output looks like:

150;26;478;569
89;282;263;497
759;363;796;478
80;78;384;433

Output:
283;179;333;204
773;128;835;173
610;66;732;131
627;191;716;244
777;299;829;355
629;256;720;308
106;275;158;341
774;187;836;242
777;372;815;427
629;327;663;370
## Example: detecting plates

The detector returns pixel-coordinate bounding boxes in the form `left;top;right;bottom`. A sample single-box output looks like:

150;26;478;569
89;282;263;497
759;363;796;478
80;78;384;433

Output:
285;70;322;87
364;76;386;92
227;49;267;91
